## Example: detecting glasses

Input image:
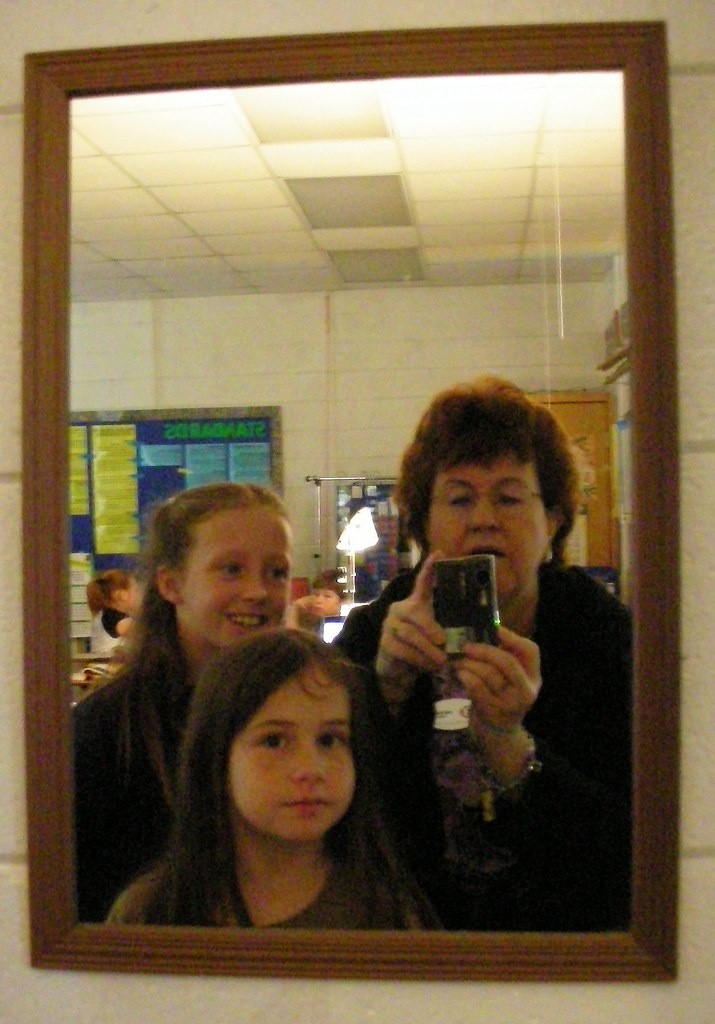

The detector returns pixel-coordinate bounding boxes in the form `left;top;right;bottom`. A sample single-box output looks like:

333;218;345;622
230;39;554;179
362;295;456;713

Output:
430;487;542;513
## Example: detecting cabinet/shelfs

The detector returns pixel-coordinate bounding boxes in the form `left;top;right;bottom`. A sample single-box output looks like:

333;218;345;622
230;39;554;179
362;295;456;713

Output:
525;390;621;574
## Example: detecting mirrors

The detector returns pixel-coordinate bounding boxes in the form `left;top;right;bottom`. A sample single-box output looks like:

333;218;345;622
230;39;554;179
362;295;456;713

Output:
22;20;681;986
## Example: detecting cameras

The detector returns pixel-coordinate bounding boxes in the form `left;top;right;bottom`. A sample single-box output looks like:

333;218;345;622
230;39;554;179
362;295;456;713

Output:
430;554;500;662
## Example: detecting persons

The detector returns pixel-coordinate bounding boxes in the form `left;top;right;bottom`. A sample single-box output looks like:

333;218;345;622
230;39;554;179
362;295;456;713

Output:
104;624;444;933
327;373;630;936
84;568;138;652
77;481;291;924
286;569;346;628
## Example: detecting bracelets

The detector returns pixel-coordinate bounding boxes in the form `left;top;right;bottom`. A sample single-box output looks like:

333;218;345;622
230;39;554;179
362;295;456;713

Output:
494;739;537;795
